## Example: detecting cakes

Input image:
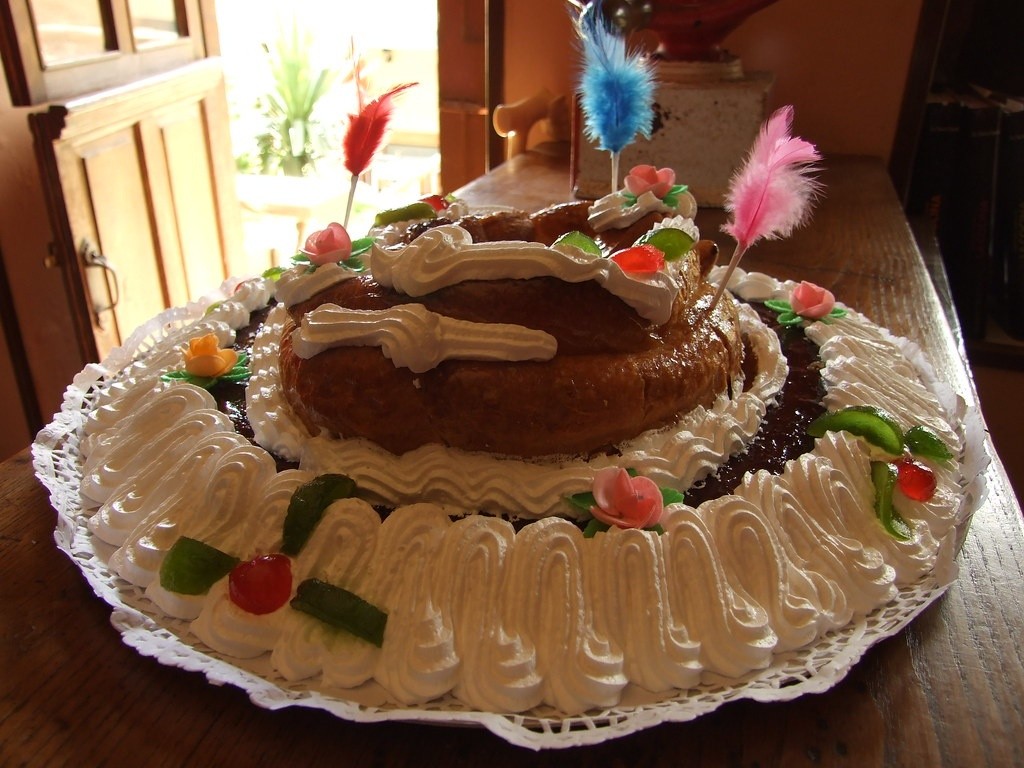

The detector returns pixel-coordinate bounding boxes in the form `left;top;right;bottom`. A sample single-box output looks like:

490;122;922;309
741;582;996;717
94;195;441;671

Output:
72;167;962;716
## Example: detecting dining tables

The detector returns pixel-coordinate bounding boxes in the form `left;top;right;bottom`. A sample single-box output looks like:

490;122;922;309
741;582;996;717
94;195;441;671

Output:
0;129;1024;768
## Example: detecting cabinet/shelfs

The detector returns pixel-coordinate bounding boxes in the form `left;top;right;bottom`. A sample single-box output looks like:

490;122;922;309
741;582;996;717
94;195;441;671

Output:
889;0;1024;373
